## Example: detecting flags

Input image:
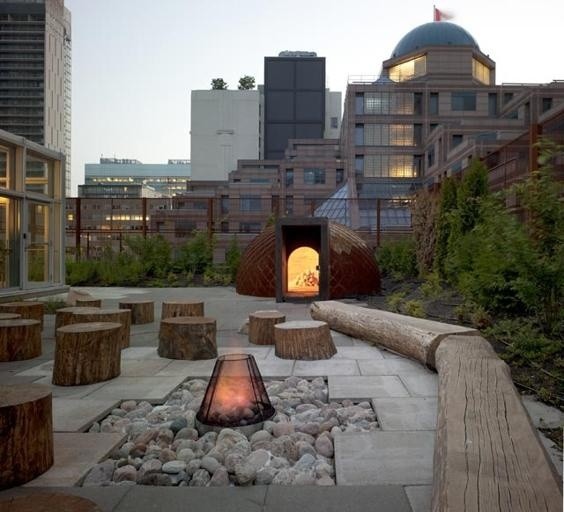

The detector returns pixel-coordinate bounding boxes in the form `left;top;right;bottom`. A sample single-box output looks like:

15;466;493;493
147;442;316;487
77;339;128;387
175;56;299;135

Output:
433;7;456;24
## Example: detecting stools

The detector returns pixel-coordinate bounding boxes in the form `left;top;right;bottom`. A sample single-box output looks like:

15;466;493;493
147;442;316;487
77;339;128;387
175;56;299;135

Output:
0;380;100;512
